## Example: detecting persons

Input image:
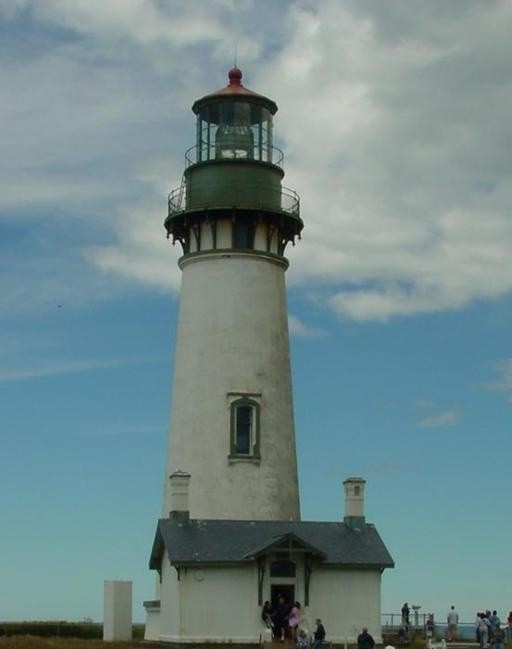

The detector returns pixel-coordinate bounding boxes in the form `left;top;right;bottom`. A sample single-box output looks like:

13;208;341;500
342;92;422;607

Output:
445;604;458;641
397;621;411;647
424;613;434;637
356;625;374;649
400;602;410;631
261;593;326;648
475;609;511;649
